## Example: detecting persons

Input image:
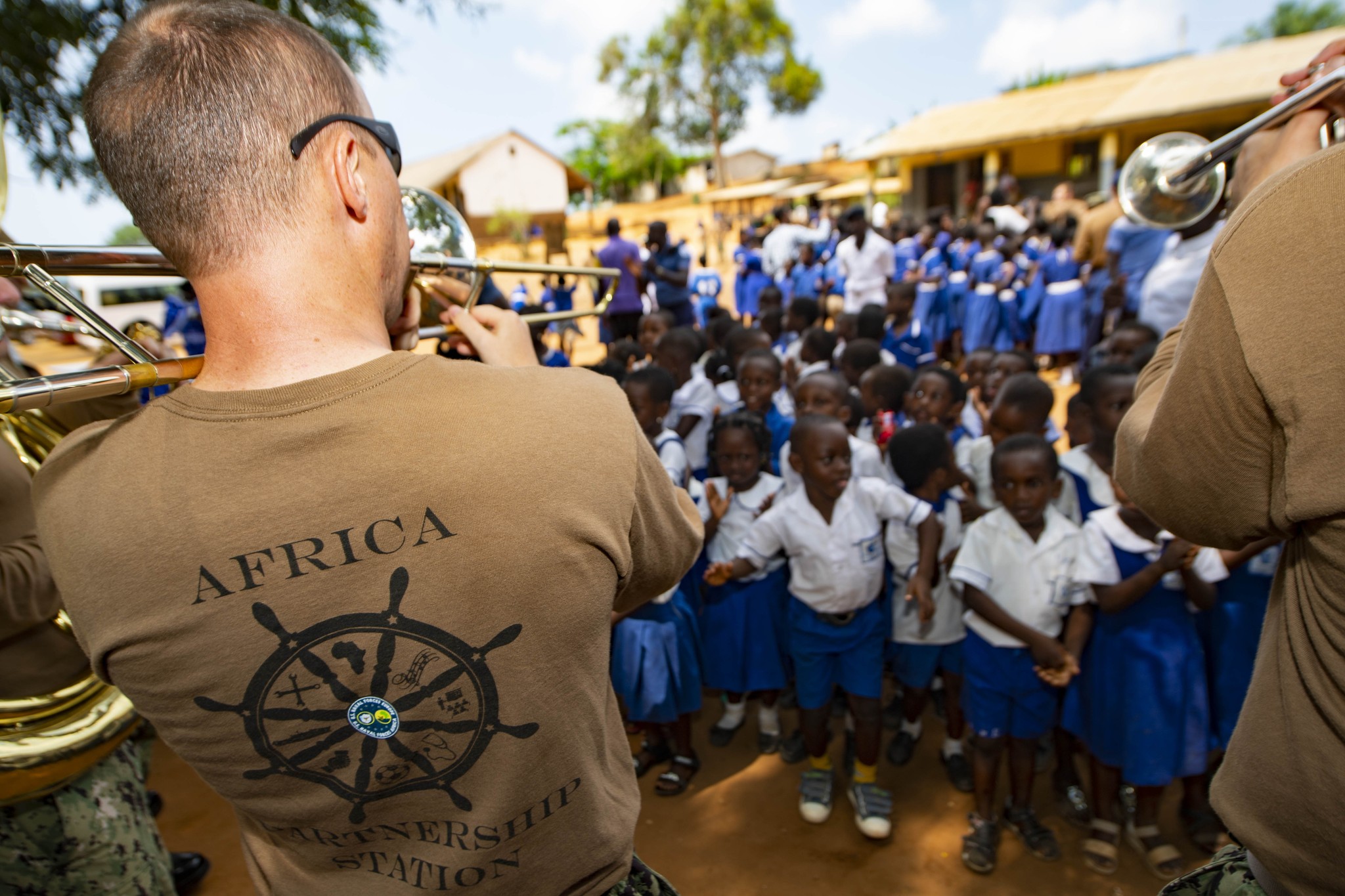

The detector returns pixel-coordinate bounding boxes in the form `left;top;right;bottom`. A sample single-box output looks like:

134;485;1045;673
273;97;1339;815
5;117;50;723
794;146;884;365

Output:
1110;33;1345;896
0;0;706;896
122;149;1285;881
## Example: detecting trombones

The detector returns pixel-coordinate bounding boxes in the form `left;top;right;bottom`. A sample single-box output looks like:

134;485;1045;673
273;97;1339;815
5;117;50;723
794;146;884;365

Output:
0;184;623;413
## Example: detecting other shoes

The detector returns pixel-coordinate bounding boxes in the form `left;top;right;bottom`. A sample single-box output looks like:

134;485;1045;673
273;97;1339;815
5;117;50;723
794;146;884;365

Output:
1178;798;1233;855
840;727;857;775
757;709;783;754
1045;766;1090;825
709;712;748;749
937;743;977;794
881;685;922;764
781;712;809;763
927;677;945;718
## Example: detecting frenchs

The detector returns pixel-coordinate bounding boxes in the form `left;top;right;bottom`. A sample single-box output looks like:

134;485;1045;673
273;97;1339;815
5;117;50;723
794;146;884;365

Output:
0;408;146;806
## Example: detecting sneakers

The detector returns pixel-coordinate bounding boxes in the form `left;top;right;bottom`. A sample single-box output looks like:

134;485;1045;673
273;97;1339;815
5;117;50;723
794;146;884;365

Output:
845;776;895;840
797;767;833;825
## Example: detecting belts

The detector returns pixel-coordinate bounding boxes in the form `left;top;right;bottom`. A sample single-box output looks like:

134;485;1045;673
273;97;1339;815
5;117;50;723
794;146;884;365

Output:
1244;847;1286;896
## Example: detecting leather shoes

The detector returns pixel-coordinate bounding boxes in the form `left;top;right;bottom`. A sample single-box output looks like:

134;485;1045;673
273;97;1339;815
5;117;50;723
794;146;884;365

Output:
167;850;210;894
145;790;166;818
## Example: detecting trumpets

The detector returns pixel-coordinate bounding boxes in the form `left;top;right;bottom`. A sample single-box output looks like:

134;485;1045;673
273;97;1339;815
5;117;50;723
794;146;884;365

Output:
1116;65;1345;232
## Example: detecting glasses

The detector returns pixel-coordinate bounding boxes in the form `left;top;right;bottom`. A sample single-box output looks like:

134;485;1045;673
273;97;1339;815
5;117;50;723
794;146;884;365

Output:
290;113;403;180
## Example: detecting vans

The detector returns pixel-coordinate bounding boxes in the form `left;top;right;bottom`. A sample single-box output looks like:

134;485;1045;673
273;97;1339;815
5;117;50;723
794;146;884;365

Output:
17;276;187;348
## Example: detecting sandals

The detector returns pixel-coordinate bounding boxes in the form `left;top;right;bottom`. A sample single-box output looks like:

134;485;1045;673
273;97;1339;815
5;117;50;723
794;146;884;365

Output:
630;739;670;778
960;809;998;875
1124;815;1189;880
999;802;1058;857
1083;810;1123;874
655;747;701;795
